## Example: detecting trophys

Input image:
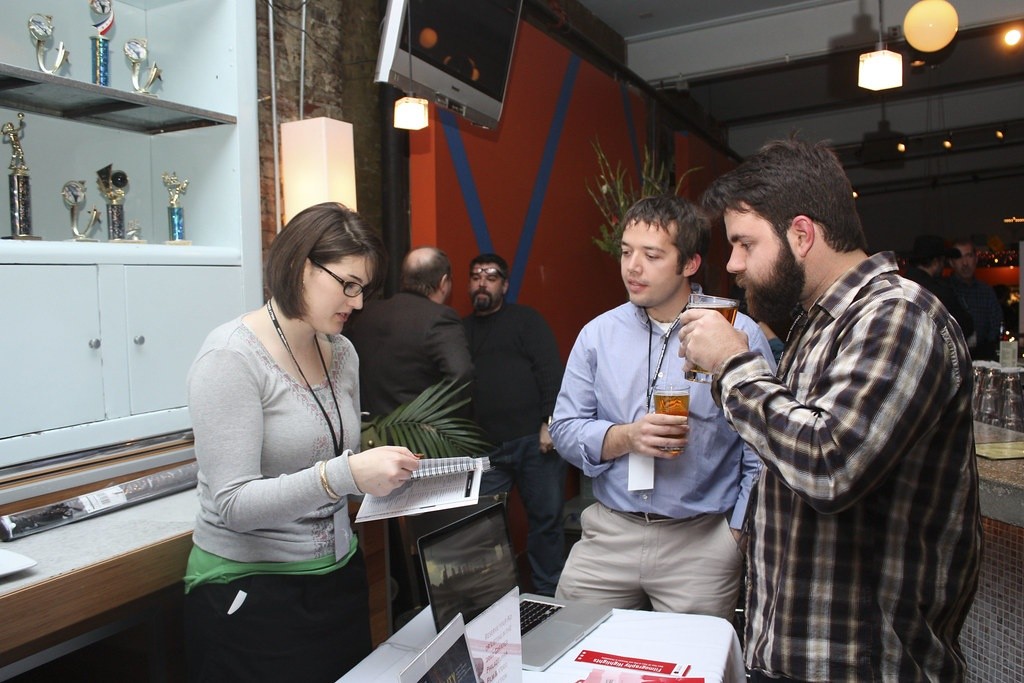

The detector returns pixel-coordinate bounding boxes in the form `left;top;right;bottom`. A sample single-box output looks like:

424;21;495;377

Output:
0;112;43;240
60;180;101;242
123;39;163;99
95;163;147;244
28;12;70;75
89;0;115;86
162;170;192;246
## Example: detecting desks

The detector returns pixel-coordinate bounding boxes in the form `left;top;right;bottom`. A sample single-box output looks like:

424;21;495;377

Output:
334;597;751;683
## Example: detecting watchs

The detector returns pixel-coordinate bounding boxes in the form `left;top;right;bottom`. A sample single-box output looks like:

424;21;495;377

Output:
542;415;552;425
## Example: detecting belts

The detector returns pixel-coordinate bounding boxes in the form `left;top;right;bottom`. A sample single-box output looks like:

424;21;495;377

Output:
634;509;679;525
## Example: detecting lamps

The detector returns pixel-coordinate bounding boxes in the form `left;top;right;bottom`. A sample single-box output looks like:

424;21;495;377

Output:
858;0;903;93
279;117;359;227
394;2;429;131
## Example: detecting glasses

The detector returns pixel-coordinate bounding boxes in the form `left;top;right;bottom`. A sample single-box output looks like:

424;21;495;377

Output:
469;266;506;281
306;255;374;300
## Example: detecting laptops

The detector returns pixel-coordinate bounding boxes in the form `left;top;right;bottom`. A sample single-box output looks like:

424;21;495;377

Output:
417;502;613;671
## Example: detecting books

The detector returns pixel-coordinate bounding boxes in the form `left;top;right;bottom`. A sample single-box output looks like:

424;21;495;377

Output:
353;457;496;523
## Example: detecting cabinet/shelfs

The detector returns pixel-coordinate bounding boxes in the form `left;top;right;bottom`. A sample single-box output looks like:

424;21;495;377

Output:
0;0;265;472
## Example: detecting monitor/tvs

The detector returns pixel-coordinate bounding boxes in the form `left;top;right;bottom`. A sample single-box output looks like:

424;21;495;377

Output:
373;0;523;131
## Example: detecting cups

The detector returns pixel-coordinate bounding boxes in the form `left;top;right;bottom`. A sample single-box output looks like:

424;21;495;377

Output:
654;384;690;456
973;367;1024;444
685;294;741;383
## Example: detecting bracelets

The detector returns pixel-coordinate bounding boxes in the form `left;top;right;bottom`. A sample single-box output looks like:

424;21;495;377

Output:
320;459;342;499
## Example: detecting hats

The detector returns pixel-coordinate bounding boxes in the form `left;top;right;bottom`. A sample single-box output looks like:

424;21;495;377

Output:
905;235;960;260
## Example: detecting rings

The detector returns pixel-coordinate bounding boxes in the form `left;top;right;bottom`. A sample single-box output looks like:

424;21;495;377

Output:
552;446;556;450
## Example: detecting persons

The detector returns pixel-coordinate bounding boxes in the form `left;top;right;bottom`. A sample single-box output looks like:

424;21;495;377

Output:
730;234;1005;367
345;247;478;424
462;253;569;598
183;203;421;683
704;128;983;683
552;194;777;625
